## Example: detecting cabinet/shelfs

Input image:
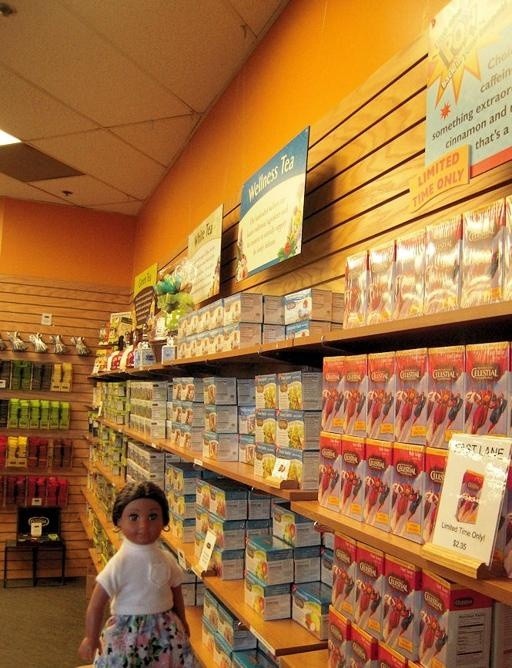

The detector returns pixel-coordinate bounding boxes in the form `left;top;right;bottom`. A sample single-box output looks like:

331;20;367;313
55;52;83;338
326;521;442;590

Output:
78;368;125;542
157;338;328;668
278;298;512;668
79;363;163;575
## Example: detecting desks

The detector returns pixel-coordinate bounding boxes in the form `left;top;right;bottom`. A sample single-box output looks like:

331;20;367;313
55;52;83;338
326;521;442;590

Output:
3;540;67;588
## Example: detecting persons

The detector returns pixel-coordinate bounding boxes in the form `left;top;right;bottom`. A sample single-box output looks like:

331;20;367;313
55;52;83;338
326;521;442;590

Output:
78;480;202;667
